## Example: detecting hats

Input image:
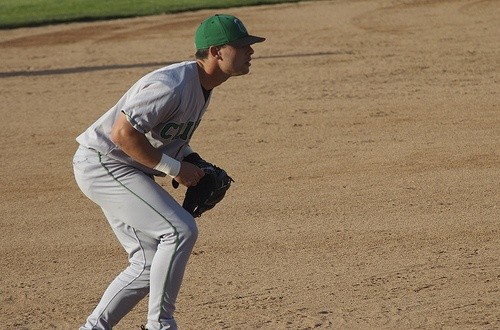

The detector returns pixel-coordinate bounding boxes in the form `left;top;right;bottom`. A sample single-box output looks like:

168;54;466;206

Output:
195;14;265;50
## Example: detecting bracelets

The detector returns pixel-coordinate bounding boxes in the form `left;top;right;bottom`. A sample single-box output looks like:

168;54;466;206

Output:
154;153;181;179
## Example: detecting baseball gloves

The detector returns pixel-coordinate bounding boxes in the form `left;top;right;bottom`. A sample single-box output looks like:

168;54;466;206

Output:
180;152;236;217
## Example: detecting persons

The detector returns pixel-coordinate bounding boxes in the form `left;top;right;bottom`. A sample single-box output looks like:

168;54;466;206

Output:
72;14;266;330
